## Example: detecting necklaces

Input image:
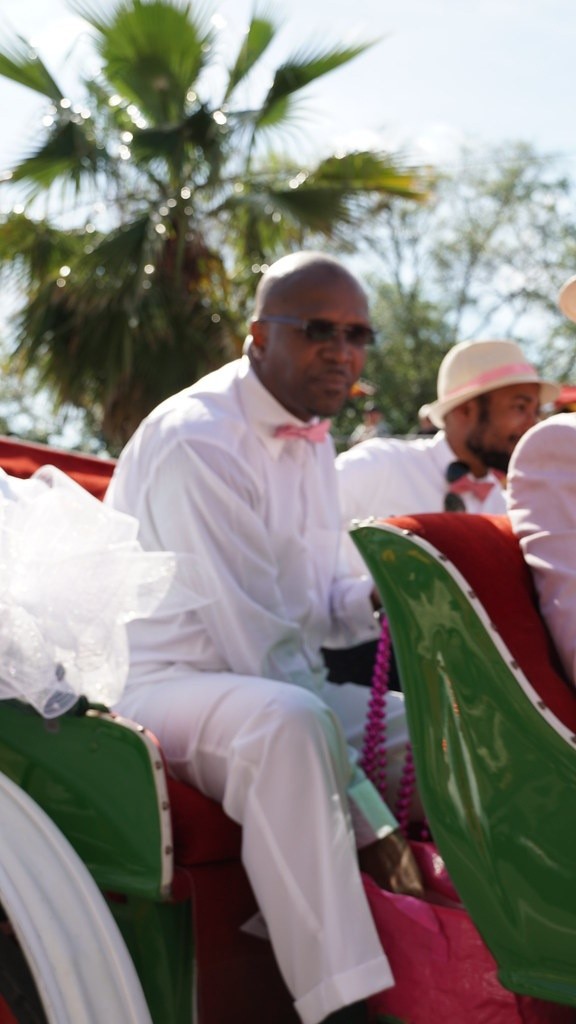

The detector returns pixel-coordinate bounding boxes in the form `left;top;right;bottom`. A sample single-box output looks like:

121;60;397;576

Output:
362;614;429;843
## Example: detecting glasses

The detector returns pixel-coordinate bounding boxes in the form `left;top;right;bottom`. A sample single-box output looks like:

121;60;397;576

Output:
259;315;374;346
445;460;472;512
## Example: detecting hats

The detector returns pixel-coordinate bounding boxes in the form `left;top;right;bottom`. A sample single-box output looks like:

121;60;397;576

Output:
427;339;559;429
560;275;576;322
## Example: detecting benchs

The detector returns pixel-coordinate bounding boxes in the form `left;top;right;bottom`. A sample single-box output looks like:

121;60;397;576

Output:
349;514;576;1011
0;696;242;903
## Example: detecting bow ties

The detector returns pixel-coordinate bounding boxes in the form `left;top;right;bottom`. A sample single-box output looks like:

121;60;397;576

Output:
450;477;494;502
274;418;331;443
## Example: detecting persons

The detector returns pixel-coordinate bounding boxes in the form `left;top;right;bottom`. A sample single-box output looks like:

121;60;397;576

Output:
415;404;438;435
335;339;559;536
346;400;391;449
101;249;423;1023
507;276;576;684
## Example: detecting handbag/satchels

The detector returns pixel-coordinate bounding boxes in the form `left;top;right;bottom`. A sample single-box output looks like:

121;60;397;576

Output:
358;837;576;1024
